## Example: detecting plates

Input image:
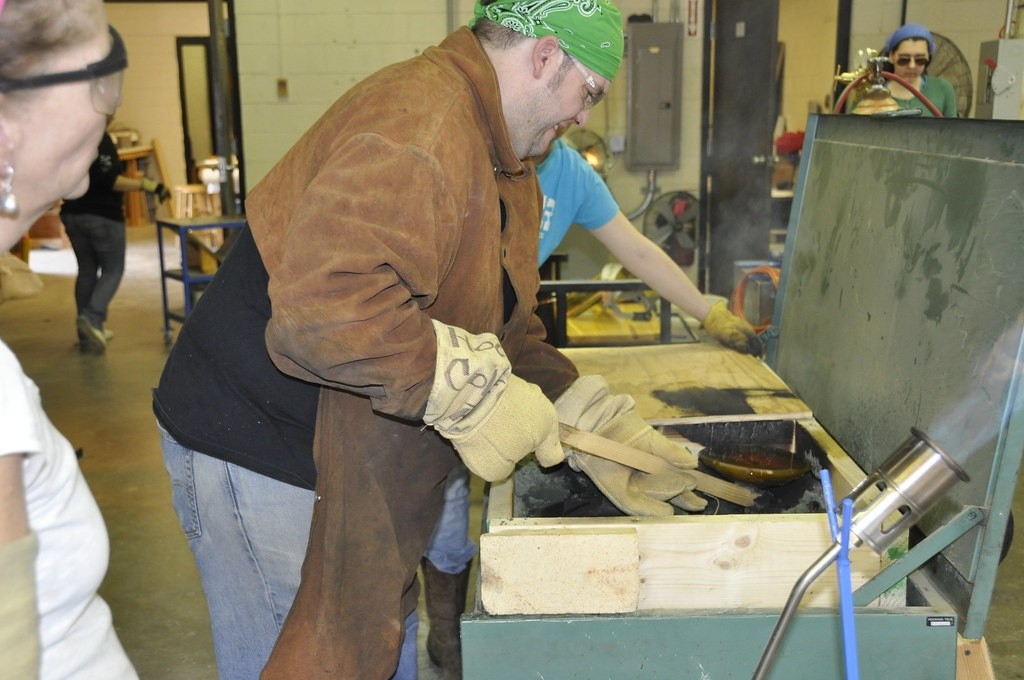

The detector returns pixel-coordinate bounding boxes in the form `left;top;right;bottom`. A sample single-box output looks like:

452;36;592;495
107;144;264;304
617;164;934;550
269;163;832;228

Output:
699;444;811;487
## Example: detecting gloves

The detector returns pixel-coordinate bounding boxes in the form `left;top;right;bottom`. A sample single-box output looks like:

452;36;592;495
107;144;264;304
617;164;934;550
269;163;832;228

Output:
554;375;708;515
140;177;173;205
422;318;566;483
704;302;763;356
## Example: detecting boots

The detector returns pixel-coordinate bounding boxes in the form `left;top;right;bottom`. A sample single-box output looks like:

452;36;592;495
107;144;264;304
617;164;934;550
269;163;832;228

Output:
420;554;473;680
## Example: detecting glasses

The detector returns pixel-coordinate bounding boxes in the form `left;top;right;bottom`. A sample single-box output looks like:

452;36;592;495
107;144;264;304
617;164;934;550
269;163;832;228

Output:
0;26;127;116
897;57;927;65
564;51;603;111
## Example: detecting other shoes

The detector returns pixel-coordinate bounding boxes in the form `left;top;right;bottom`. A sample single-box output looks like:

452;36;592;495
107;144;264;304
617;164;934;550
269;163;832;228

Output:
102;328;114;341
77;315;107;352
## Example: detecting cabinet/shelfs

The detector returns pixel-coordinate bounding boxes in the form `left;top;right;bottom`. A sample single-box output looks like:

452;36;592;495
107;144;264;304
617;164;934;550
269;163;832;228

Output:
156;215;247;346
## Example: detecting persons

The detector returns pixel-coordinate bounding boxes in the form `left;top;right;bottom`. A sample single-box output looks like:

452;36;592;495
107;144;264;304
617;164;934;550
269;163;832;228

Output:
849;22;957;119
420;133;764;680
0;0;143;680
149;0;705;680
58;112;170;353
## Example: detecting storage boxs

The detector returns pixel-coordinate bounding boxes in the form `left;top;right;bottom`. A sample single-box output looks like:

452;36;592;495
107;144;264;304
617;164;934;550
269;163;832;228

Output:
483;340;909;615
458;114;1023;680
